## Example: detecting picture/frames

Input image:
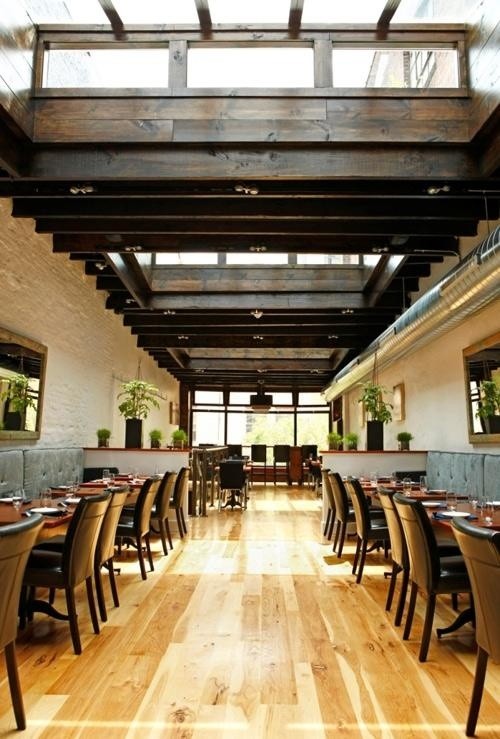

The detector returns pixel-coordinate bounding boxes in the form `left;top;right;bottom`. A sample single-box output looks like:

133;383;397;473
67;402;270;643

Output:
392;383;405;422
358;400;365;427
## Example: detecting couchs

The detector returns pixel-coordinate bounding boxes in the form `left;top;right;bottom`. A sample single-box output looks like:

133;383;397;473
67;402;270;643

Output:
423;452;499;501
1;448;84;499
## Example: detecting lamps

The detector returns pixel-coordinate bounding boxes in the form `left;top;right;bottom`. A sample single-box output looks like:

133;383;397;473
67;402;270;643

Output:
250;374;272;414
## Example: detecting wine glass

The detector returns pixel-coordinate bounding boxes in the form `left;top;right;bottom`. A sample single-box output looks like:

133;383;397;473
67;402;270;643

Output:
302;452;321;470
0;464;183;519
341;469;500;529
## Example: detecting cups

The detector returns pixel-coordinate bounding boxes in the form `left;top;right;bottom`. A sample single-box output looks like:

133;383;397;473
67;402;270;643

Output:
220;454;249;461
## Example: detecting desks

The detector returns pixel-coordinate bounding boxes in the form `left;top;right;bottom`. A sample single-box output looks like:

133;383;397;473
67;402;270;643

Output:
1;474;162;546
344;474;499;548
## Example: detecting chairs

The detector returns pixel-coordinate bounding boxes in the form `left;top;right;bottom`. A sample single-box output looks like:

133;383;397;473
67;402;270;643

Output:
118;470;178;556
321;468;354;541
346;479;391;584
328;471;386;559
116;476;162;581
18;491;111;656
32;484;131;622
190;444;322;518
392;492;477;663
449;516;500;738
374;486;465;628
0;513;45;729
168;467;189;539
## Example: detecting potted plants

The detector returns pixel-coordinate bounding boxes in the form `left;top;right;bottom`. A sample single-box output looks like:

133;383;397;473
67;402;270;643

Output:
149;430;162;449
98;429;111;448
475;382;500;434
343;432;358;451
397;432;413;451
0;373;38;430
327;432;342;450
117;380;160;448
352;381;393;450
173;430;188;448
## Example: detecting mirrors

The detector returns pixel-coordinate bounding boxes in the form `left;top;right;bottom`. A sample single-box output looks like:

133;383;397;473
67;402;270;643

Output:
0;327;48;439
462;332;500;443
